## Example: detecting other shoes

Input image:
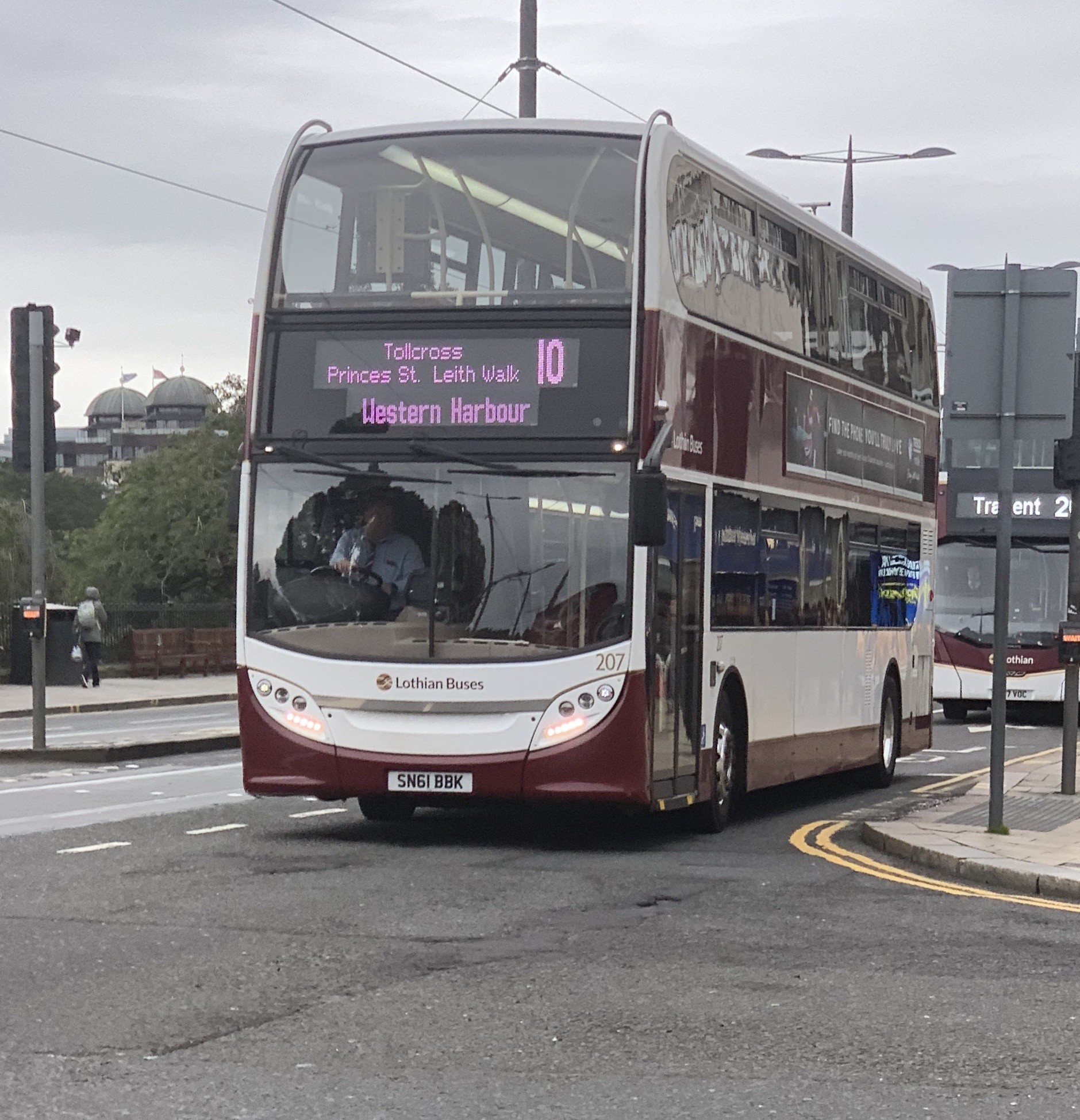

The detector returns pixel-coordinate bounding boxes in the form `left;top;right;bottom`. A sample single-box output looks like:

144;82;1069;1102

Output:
79;673;89;689
93;683;100;688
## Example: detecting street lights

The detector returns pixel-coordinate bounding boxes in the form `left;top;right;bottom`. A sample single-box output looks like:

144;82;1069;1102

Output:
490;560;562;640
453;488;525;640
740;132;961;238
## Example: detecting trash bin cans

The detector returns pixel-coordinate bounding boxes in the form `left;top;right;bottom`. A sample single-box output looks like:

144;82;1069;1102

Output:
10;604;83;686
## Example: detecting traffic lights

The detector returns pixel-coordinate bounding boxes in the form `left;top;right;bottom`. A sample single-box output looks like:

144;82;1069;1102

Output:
10;302;62;474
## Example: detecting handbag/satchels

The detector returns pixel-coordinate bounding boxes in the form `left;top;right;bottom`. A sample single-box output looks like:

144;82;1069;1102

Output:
78;599;95;628
71;644;84;663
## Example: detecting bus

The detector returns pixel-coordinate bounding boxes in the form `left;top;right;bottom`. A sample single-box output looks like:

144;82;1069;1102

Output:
226;109;943;836
932;462;1079;726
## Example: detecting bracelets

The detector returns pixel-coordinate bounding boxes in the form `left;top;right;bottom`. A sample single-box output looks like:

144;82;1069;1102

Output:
391;583;398;597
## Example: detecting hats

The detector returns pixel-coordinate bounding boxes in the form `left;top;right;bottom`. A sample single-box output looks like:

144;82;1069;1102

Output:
86;586;100;598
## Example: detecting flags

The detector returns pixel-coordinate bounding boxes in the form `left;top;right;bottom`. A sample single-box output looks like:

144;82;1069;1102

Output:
153;370;168;381
123;373;136;382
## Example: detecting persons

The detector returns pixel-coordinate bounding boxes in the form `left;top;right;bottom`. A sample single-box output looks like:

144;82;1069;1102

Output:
73;586;109;688
328;487;426;611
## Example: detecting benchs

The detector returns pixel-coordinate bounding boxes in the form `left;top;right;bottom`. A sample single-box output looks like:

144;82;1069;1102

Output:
192;626;236;673
131;625;214;680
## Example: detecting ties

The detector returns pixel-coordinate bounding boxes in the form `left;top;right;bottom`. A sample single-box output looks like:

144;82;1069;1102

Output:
359;542;376;583
